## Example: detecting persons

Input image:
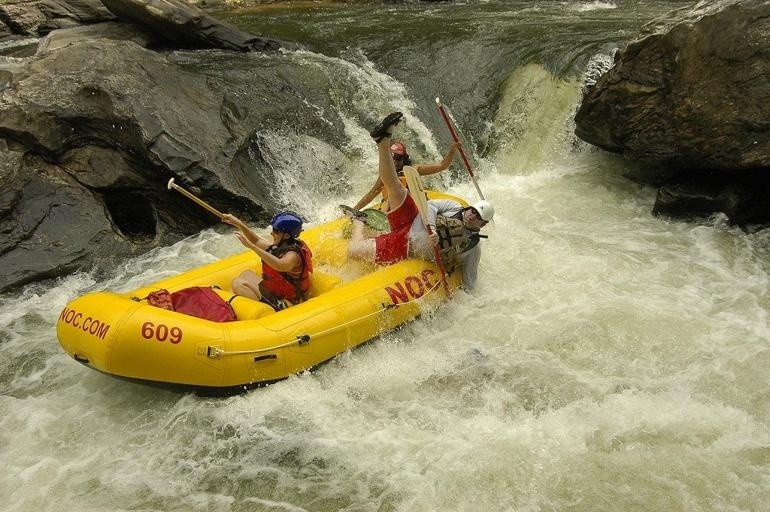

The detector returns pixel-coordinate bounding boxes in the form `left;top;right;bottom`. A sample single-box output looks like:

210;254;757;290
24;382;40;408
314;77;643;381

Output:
219;210;313;313
338;111;497;298
349;140;462;234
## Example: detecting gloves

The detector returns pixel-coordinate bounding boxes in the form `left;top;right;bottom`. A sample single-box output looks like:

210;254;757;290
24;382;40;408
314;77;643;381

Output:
426;226;440;246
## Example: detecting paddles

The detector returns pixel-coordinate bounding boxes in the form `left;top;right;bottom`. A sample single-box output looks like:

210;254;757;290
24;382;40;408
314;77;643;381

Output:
403;166;451;299
167;178;274;245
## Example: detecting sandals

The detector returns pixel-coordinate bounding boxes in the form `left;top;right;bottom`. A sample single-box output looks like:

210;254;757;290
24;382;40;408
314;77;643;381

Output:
370;112;403;145
339;205;368;224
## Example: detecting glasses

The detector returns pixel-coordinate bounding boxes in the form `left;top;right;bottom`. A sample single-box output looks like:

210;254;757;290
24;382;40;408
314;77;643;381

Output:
471;208;481;221
393;155;404;160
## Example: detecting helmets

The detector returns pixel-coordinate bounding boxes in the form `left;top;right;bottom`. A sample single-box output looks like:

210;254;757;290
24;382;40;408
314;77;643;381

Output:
470;200;494;223
389;142;405;155
269;211;303;239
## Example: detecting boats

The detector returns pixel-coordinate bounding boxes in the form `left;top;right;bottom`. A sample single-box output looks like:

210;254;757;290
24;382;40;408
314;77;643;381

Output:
56;191;472;399
